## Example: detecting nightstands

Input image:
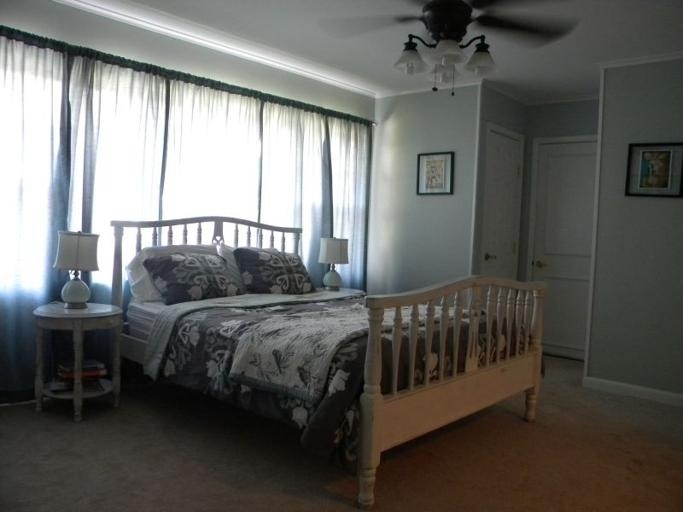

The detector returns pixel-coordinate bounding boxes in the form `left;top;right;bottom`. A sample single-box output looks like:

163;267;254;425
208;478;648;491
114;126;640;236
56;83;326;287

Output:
32;302;124;423
317;288;365;296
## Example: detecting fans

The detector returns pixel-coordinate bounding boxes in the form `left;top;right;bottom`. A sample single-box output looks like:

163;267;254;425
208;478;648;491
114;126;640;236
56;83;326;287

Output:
322;0;579;48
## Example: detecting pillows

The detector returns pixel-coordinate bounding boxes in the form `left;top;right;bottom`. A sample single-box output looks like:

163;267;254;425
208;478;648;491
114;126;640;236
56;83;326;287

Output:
125;243;316;305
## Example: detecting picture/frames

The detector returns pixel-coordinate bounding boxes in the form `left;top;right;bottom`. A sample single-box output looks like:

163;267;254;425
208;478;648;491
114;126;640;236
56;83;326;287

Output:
624;143;683;199
416;151;454;195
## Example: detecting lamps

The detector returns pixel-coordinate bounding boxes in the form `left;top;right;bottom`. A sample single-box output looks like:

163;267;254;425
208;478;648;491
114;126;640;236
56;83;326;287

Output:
318;237;349;291
52;230;100;310
392;34;498;96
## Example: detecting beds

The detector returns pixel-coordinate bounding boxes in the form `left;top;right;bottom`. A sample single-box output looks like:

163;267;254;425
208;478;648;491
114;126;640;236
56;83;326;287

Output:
109;216;547;510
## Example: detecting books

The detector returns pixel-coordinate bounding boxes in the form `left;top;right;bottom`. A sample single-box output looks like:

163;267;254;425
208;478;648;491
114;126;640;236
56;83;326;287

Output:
50;358;107;394
